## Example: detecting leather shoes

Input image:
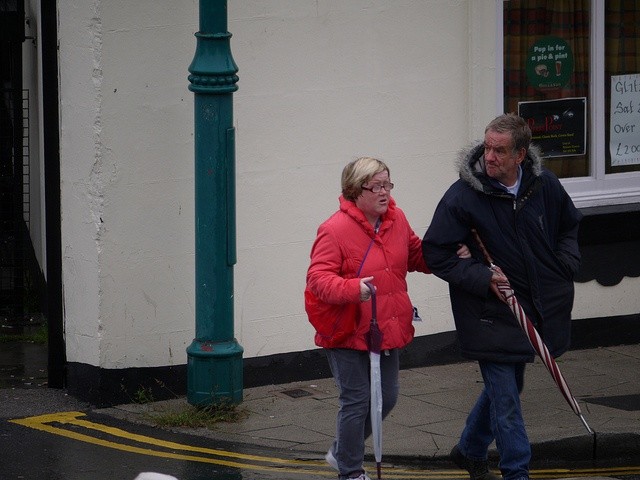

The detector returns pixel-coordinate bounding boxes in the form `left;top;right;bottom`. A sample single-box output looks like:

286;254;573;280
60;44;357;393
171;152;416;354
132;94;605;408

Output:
448;444;502;480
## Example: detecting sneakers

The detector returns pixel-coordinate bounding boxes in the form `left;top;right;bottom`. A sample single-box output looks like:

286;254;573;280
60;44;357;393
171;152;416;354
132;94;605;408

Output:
345;471;371;480
325;450;341;471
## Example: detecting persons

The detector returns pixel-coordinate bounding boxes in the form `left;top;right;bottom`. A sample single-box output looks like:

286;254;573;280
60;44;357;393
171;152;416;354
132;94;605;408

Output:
304;157;472;480
422;113;583;480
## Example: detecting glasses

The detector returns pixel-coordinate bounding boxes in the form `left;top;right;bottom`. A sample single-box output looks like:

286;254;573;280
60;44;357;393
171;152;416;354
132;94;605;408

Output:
361;182;394;193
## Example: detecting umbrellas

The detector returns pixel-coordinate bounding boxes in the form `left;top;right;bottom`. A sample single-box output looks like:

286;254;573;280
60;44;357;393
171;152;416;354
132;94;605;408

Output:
471;229;592;433
363;282;384;480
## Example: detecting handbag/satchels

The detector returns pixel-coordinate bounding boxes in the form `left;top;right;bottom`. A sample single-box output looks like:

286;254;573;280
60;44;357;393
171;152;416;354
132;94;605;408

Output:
304;214;382;347
